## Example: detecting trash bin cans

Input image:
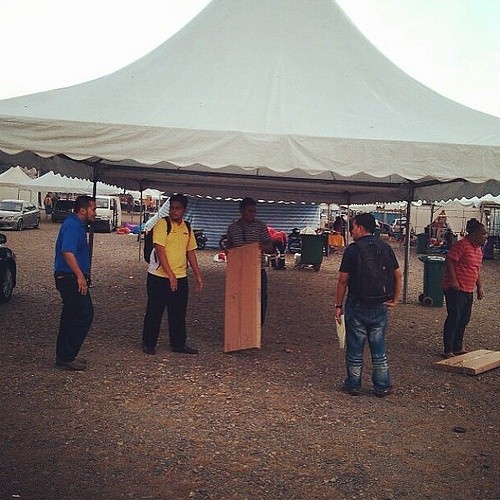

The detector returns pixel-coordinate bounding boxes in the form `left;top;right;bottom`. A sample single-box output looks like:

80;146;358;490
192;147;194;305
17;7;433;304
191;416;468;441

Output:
300;235;325;270
415;233;428;254
374;228;381;238
419;255;445;307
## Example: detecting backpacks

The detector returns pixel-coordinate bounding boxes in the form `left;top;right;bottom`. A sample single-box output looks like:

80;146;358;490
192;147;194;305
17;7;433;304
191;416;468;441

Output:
348;242;394;304
144;217;191;263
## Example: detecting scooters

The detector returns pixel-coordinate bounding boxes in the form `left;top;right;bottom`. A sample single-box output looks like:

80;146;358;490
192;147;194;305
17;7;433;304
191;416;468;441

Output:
193;227;207;250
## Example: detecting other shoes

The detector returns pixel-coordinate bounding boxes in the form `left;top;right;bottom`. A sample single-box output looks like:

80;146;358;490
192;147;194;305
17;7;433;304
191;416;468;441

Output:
56;362;86;371
341;385;359;396
74;356;87;363
453;350;469;355
375;386;393;397
143;344;155;354
441;352;456;359
173;343;198;354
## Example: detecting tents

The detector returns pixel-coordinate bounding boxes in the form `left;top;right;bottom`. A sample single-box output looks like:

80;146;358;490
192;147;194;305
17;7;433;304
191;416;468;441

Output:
0;0;500;304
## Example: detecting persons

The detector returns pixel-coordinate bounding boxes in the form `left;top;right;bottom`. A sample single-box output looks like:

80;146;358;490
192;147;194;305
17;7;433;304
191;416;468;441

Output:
442;218;488;359
45;195;52;214
141;194;203;355
226;197;274;326
54;195;97;370
48;192;57;204
336;213;402;398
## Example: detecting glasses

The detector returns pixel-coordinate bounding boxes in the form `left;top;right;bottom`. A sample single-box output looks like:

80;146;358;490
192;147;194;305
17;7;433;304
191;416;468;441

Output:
481;234;488;237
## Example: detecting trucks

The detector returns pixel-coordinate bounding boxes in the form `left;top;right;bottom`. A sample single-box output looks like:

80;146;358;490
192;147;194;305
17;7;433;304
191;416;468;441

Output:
409;200;500;253
87;194;121;232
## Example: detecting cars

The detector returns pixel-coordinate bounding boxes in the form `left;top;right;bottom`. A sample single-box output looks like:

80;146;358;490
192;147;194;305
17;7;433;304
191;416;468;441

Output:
52;198;75;221
0;232;18;301
389;218;405;238
0;198;42;230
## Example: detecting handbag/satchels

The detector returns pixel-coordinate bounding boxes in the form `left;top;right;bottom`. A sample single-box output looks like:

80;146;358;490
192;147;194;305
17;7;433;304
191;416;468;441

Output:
335;314;346;349
